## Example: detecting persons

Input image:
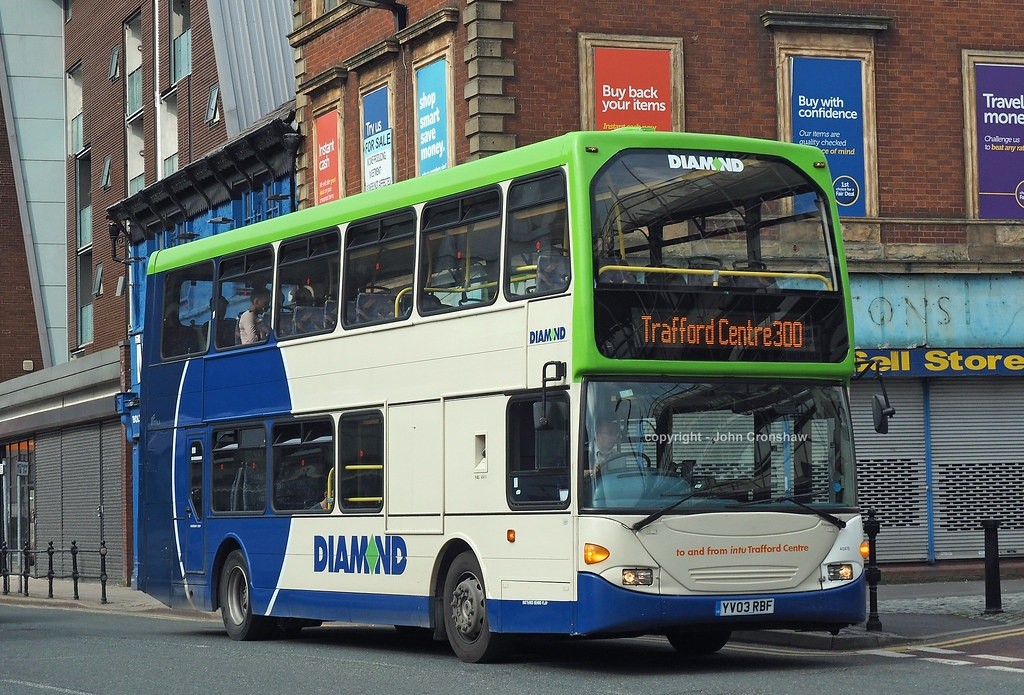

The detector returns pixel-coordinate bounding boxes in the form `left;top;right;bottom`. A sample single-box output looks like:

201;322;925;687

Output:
295;285;323;308
202;296;237;349
275;458;334;515
583;420;627;478
239;286;271;345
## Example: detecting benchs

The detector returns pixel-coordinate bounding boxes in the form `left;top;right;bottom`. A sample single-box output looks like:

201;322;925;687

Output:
163;245;570;359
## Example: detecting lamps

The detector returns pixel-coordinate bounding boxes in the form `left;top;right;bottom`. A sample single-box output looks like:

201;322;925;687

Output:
122;257;146;263
23;359;34;373
207;217;234;224
175;233;200;239
266;194;291;201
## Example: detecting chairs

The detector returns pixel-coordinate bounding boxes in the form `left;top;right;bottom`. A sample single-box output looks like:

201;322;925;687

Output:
642;264;687;286
686;255;734;286
597;258;639;284
731;260;780;290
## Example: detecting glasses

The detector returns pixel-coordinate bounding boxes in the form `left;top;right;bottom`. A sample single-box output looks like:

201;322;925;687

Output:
598;430;621;437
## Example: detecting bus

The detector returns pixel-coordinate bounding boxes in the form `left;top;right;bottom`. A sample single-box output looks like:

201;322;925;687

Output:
137;127;895;664
